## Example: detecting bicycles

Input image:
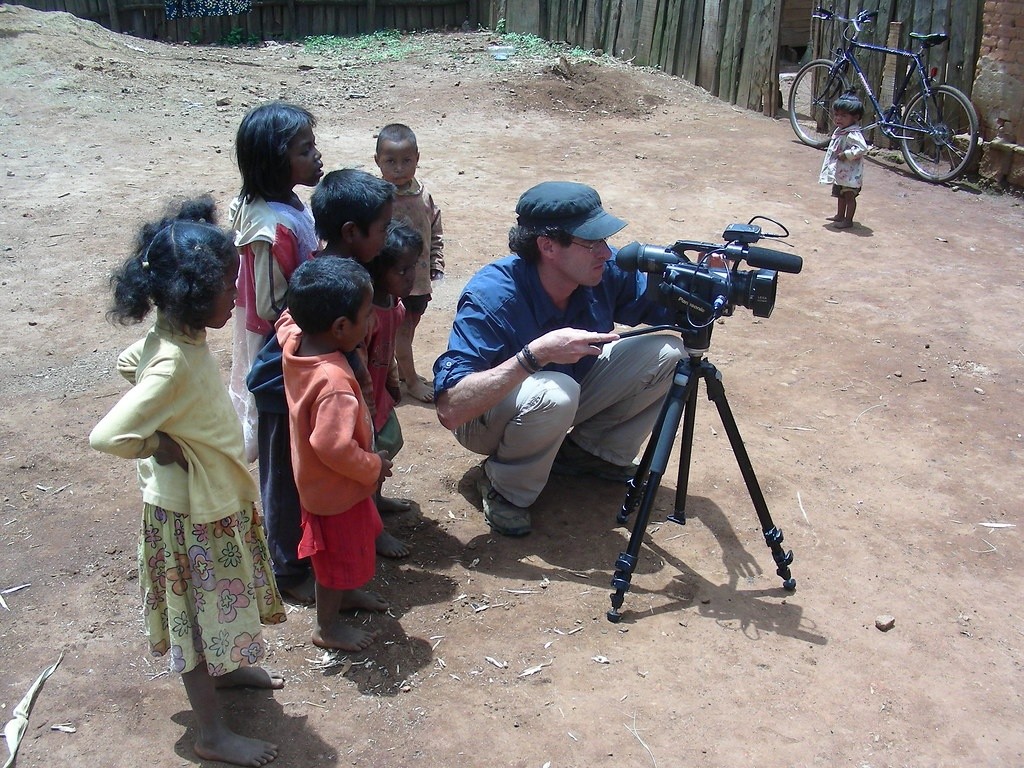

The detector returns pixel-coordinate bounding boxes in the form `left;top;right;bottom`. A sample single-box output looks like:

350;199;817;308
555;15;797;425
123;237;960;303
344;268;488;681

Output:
787;5;978;182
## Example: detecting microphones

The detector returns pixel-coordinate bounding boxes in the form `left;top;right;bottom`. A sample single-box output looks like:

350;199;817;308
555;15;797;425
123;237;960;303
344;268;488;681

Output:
675;240;803;274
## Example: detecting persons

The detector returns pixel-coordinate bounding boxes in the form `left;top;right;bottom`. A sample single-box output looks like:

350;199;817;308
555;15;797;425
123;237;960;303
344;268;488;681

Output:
432;181;730;539
89;195;287;767
273;256;392;650
230;102;444;607
818;85;868;228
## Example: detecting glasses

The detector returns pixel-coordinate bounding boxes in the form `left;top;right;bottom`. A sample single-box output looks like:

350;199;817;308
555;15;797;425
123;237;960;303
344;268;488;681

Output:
568;237;609;254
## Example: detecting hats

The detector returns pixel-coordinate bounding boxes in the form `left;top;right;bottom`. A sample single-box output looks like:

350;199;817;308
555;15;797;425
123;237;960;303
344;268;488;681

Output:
516;181;628;241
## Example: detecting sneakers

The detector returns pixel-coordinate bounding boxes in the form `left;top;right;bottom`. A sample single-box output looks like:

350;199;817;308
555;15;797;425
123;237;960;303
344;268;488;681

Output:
475;455;532;535
552;433;649;482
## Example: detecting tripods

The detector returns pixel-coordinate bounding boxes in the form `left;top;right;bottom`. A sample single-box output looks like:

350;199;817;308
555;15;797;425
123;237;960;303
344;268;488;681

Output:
586;313;796;623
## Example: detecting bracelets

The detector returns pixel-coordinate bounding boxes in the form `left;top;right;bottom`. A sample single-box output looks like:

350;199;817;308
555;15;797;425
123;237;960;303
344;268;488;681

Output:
516;344;544;374
